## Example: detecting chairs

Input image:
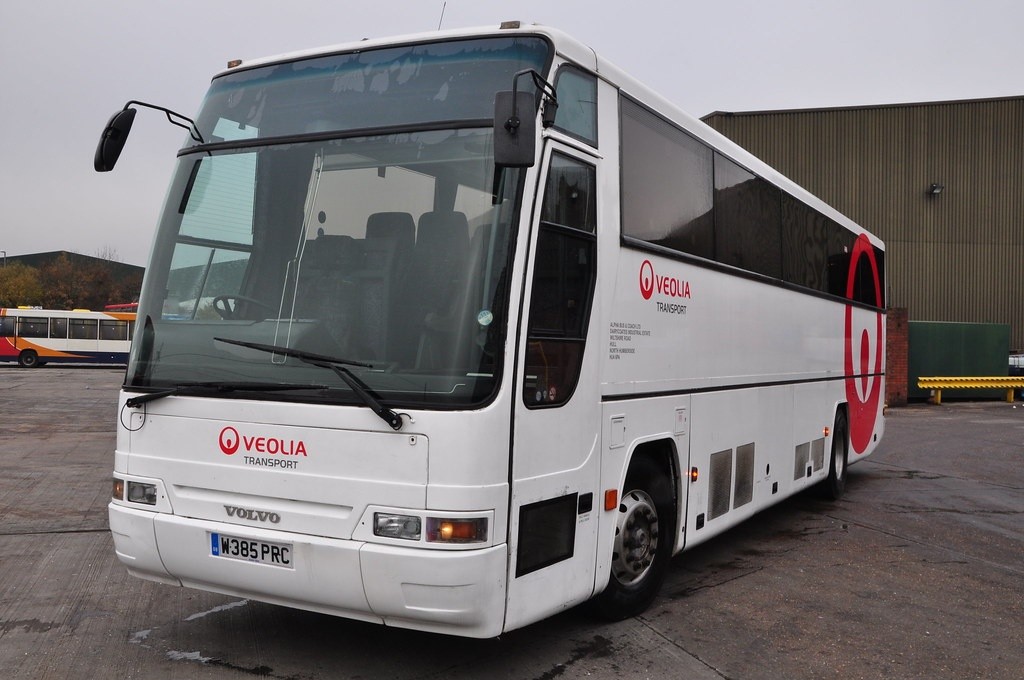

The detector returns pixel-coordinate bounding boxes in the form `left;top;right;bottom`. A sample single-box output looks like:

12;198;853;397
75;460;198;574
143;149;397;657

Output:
289;207;504;354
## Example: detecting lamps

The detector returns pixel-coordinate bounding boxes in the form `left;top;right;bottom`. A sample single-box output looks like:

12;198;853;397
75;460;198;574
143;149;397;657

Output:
929;183;945;194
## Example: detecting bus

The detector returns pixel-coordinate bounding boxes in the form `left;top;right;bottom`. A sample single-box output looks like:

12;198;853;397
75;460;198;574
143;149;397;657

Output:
93;23;886;642
103;303;139;312
1;306;137;367
